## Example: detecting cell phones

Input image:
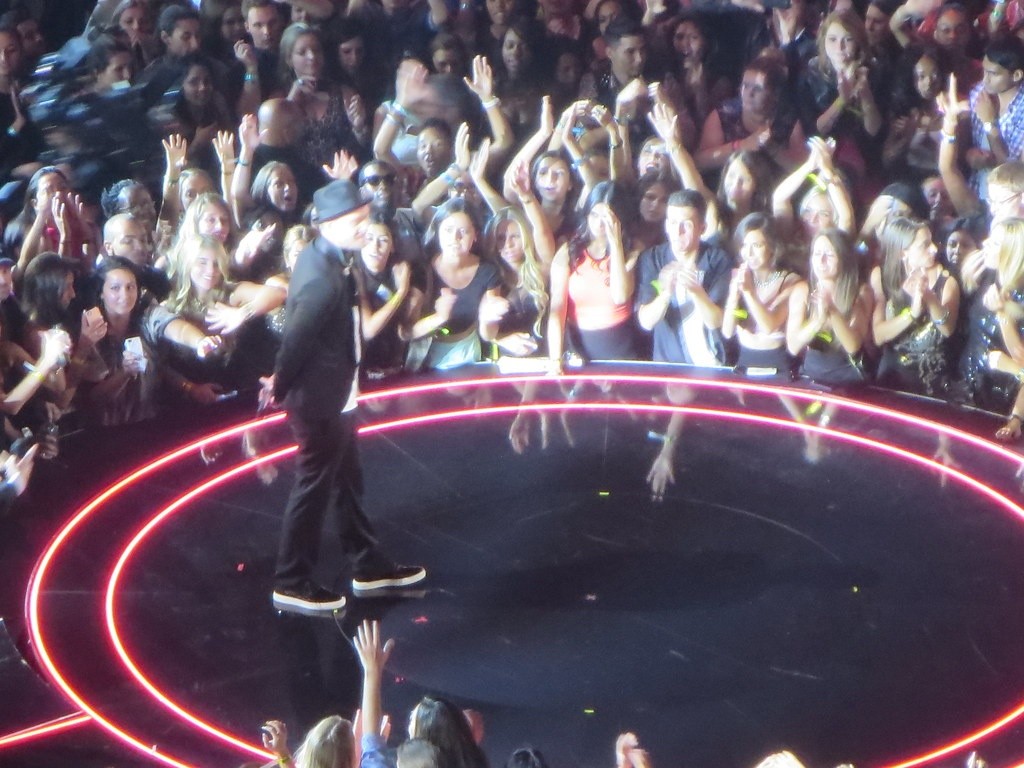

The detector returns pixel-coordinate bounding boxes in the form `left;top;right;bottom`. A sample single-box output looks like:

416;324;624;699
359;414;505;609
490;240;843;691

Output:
124;336;145;373
85;307;102;327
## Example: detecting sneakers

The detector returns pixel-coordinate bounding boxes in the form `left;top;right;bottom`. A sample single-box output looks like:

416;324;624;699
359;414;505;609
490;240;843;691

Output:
352;562;426;590
273;581;346;610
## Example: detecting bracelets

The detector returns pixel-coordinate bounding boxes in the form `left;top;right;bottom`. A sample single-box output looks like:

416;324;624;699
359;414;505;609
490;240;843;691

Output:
607;138;623;149
181;379;194;393
244;72;259;81
440;171;455;186
731;138;741;151
276;753;291;765
237;158;252;167
385;113;402;128
833;97;846;112
613;114;630;123
81;237;97;245
569;153;589;169
481;95;502;110
222;171;233;176
163;174;180;185
65;360;89;385
449;162;464;176
930;308;952;325
938;128;960;144
390;101;410;117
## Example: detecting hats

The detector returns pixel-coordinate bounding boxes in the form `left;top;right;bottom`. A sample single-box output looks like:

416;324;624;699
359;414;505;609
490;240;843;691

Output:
311;179;373;224
878;182;926;217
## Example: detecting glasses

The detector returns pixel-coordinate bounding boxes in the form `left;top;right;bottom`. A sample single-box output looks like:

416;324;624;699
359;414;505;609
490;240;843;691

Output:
359;174;395;187
985;192;1022;207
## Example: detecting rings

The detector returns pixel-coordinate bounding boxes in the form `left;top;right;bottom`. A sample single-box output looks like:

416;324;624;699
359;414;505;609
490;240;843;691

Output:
297;78;305;85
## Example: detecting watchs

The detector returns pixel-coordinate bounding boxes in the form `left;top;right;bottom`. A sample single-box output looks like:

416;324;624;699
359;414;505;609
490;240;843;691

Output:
980;121;999;132
1008;414;1024;427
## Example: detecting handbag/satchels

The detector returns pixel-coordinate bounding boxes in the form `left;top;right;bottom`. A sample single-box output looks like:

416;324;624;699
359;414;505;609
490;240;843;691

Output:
404;265;434;374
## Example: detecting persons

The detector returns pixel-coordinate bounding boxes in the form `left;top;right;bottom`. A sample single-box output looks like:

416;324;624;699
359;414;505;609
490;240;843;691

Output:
259;178;428;614
0;0;1024;768
